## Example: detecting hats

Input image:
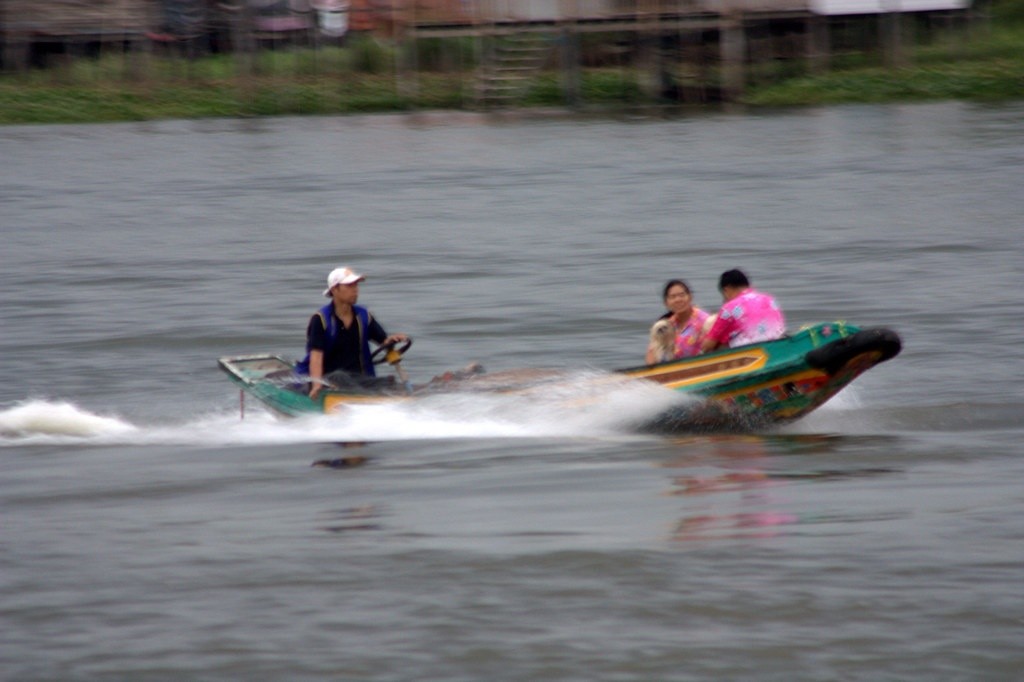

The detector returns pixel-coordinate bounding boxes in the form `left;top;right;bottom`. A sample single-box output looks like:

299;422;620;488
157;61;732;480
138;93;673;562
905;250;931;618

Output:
323;269;365;297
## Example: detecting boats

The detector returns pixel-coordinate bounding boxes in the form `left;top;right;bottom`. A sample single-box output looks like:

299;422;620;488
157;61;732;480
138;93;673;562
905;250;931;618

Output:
215;317;906;431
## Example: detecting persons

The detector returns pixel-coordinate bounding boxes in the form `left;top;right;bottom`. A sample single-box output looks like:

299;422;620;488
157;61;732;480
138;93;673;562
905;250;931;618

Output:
645;269;786;365
296;267;408;401
